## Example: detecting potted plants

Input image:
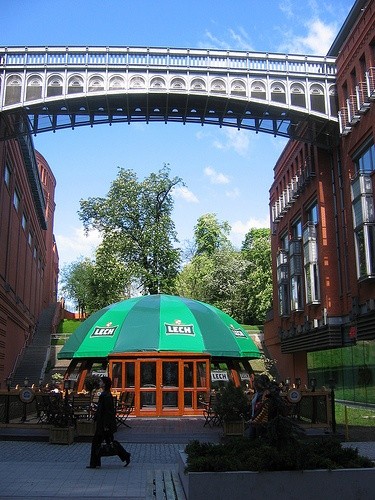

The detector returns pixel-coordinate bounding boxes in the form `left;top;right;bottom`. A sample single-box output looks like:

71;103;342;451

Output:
75;374;103;436
216;380;250;436
49;410;77;444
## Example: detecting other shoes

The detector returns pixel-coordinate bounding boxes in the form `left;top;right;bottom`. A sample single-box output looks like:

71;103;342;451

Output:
124;453;131;467
86;464;101;469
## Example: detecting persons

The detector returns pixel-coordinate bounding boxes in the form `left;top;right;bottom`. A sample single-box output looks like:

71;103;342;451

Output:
246;373;287;425
86;377;131;469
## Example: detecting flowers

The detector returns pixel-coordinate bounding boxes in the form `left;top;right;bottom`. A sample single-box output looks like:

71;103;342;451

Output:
45;373;65;395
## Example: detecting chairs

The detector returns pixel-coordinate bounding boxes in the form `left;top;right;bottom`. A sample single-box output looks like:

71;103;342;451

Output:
200;394;298;428
38;394;135;430
